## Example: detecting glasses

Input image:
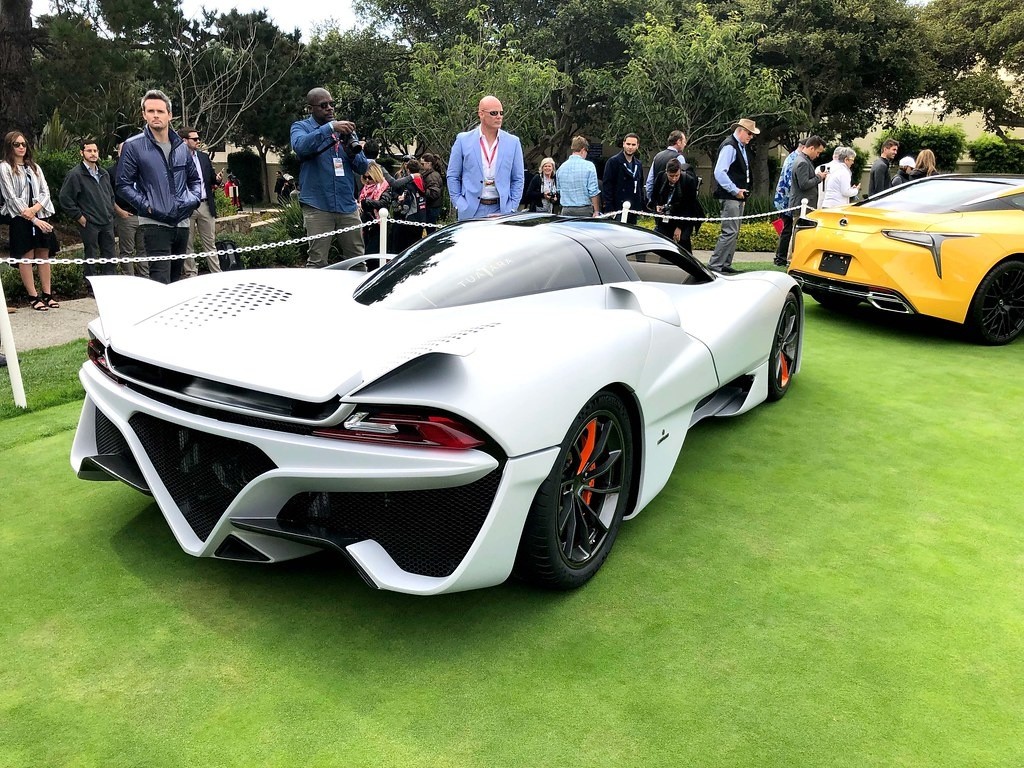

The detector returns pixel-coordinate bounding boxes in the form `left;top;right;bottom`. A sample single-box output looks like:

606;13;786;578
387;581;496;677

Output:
744;131;754;136
188;137;201;141
482;111;504;116
13;142;27;148
907;166;913;170
308;101;336;109
626;143;636;146
421;163;429;166
847;157;854;161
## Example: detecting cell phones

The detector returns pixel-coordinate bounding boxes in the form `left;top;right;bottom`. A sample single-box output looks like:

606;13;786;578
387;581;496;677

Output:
743;191;752;199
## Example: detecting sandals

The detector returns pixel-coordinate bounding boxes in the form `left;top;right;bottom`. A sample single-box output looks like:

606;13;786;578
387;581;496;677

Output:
29;294;48;310
41;291;59;308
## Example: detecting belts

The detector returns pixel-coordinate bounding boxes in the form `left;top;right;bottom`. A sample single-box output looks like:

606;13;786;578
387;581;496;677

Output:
480;198;500;205
200;199;208;202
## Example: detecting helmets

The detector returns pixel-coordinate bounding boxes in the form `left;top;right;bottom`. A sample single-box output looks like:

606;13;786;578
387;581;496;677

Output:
363;174;372;180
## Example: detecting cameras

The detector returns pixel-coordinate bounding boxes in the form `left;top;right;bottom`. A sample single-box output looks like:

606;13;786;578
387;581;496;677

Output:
661;205;672;215
339;129;362;155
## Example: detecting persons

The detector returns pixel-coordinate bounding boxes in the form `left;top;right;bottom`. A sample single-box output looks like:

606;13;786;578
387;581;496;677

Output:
706;118;760;274
178;127;224;278
601;133;644;227
212;174;243;211
891;156;916;187
645;130;706;264
114;90;202;285
290;87;368;272
446;95;524;221
868;138;899;198
774;135;827;268
556;134;601;218
814;147;861;209
106;142;149;279
909;149;939;180
354;140;444;272
59;139;118;299
0;132;60;311
517;157;560;214
274;170;300;210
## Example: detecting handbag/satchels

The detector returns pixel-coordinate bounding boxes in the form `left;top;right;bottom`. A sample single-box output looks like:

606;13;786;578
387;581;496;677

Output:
772;219;784;236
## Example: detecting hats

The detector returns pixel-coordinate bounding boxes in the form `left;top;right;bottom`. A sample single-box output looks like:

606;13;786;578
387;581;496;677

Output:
730;119;760;134
364;141;379;159
899;156;916;168
798;139;808;145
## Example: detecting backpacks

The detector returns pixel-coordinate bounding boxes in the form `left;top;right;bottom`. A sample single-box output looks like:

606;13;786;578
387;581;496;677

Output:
215;240;242;271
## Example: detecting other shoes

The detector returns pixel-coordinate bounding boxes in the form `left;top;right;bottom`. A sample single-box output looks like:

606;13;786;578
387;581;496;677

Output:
773;261;789;268
721;267;743;275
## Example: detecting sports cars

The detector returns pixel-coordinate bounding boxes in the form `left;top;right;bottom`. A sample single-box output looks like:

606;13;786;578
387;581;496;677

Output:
786;170;1024;345
67;209;806;600
